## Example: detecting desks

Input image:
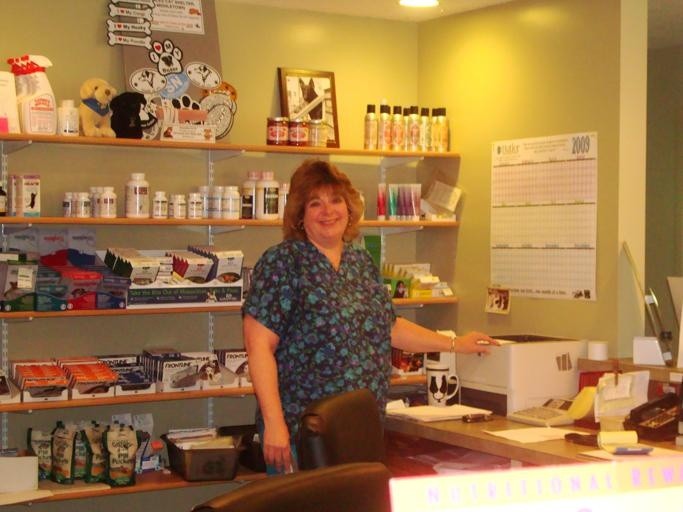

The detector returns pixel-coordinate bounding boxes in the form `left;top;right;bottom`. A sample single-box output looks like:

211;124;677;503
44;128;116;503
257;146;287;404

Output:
375;395;683;467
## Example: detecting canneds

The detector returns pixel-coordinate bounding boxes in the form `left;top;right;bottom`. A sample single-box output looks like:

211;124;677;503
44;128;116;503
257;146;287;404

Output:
267;117;327;147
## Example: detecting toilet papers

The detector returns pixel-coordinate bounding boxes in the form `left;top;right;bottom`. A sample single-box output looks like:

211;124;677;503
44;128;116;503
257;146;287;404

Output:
587;341;609;360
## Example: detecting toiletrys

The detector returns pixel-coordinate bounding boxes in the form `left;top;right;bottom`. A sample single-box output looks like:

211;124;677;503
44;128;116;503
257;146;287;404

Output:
377;183;422;221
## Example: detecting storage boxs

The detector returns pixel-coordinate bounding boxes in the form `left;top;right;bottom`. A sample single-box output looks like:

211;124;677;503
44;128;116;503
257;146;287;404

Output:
158;427;247;482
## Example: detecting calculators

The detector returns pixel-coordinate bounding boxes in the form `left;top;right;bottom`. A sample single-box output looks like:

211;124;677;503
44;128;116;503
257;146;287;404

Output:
509;399;575;428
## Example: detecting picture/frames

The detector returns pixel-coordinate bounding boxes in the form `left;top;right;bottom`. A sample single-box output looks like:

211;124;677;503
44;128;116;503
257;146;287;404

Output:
275;65;341;149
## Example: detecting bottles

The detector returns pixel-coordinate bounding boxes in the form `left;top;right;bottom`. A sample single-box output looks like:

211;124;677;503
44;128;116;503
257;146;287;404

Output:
58;99;80;138
62;168;288;223
364;104;450;153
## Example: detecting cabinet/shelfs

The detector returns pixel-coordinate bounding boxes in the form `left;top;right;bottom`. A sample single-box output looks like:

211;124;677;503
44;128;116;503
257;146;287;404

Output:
0;130;464;504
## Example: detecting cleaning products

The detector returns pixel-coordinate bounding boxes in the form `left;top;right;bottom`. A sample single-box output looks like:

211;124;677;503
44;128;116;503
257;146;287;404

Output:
363;105;450;152
241;169;280;217
6;53;57;133
28;418;137;486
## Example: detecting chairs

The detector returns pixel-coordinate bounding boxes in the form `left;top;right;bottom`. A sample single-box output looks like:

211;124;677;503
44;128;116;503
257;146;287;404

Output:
190;456;393;512
289;385;387;471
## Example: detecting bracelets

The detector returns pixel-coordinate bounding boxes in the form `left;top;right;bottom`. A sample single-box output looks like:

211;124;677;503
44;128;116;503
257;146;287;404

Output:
450;336;455;353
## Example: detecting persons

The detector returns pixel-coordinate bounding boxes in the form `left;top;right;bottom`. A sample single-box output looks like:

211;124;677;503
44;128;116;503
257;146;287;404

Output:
241;160;500;478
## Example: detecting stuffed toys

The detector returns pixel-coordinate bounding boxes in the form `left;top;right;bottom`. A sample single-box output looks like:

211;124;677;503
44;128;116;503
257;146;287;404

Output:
109;91;146;138
76;79;117;138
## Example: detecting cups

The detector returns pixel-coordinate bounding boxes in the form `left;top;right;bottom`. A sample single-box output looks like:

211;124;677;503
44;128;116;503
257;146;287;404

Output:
587;340;610;362
425;364;460;408
